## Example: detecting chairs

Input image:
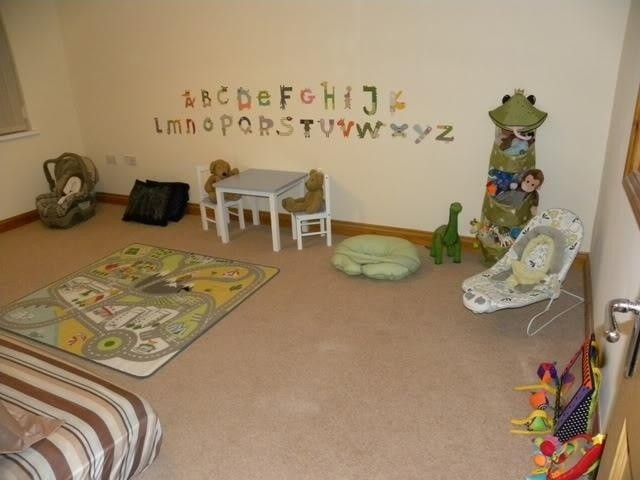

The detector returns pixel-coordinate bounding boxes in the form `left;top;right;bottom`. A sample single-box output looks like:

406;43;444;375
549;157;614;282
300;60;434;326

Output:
290;168;332;251
194;164;246;232
461;207;586;338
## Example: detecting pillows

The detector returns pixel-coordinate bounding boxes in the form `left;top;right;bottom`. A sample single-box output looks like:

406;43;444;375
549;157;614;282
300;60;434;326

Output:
331;233;423;282
121;178;191;228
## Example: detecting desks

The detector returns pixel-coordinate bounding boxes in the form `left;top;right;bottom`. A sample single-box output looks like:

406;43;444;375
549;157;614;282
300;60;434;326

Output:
212;168;309;253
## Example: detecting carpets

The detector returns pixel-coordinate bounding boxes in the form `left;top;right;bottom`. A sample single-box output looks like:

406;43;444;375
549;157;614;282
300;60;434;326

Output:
0;242;281;378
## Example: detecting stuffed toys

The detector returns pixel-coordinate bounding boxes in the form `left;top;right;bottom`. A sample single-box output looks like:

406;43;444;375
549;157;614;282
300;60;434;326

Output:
282;170;325;215
204;159;241;204
469;87;545;265
